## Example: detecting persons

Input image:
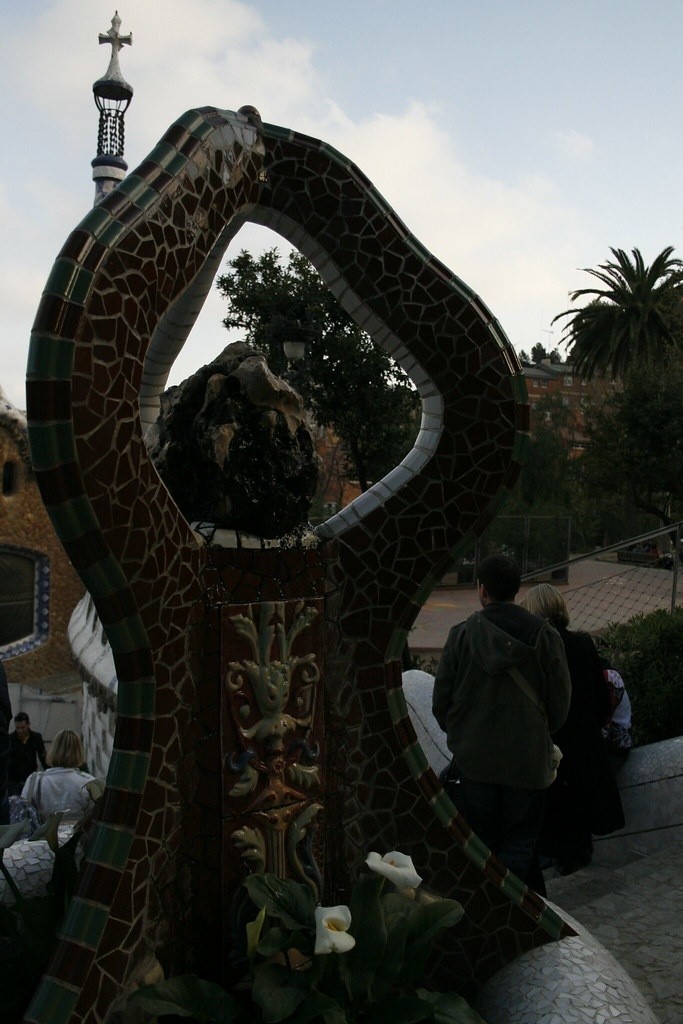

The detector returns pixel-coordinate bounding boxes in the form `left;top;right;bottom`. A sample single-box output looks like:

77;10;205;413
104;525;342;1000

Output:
0;712;104;822
433;554;633;903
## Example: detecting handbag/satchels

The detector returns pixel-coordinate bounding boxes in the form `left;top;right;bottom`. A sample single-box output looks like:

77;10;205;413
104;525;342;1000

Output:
438;755;455;794
587;740;619;776
7;771;42;841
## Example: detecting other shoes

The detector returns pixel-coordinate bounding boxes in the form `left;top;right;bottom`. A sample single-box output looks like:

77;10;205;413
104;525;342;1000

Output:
538;855;556;871
555;856;592;875
